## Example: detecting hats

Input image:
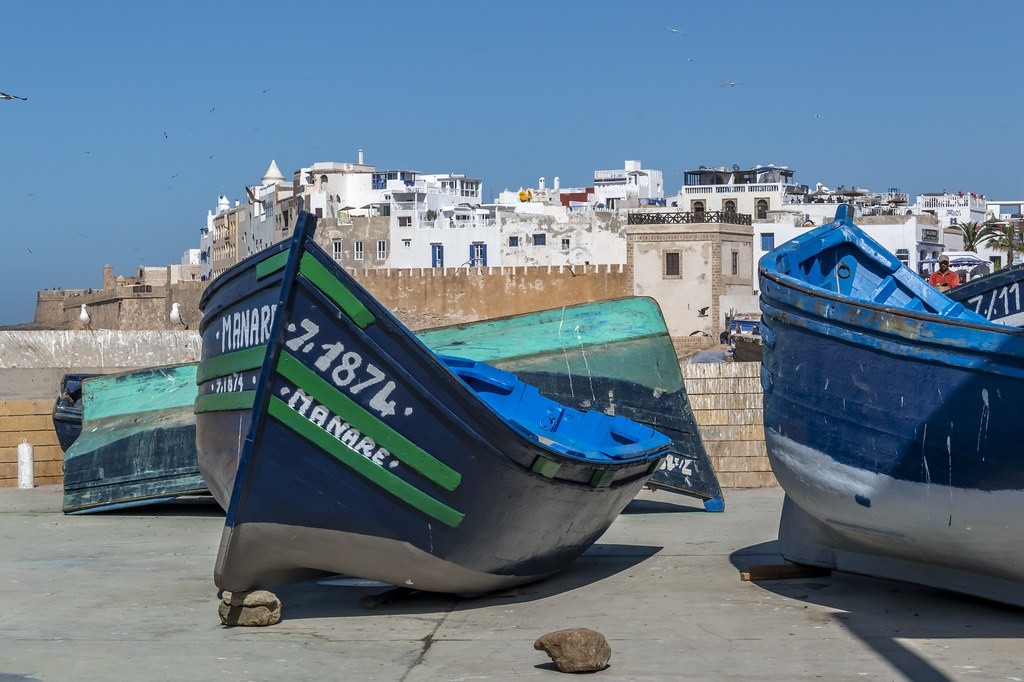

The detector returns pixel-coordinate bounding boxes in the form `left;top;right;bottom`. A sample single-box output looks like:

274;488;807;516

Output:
938;255;949;263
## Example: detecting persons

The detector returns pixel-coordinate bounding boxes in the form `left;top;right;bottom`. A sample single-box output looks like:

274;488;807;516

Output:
520;188;532;203
930;255;959;292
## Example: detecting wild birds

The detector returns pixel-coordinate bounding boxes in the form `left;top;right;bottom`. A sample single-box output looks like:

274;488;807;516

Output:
665;28;745;87
80;303;187;331
0;88;321;290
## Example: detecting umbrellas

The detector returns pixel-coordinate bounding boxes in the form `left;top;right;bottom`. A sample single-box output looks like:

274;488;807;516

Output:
918;257;990;281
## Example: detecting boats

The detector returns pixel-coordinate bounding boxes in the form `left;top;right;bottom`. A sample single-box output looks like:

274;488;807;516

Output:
196;207;673;599
728;263;1024;364
756;204;1023;610
49;295;729;518
